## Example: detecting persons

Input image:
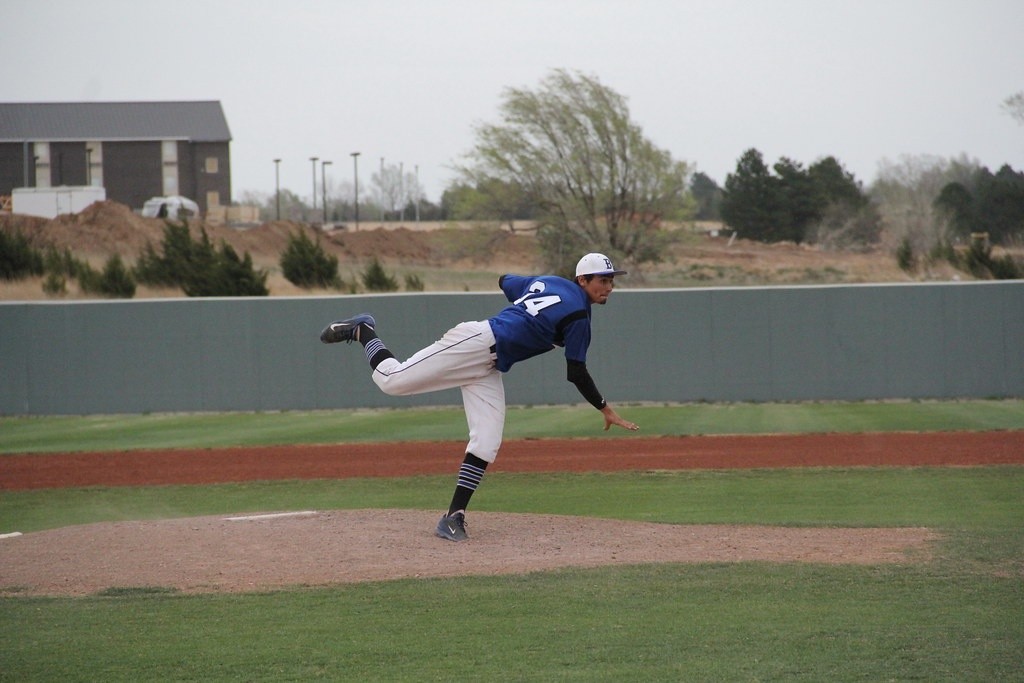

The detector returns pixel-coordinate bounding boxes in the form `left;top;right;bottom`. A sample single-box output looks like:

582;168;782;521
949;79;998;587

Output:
320;252;640;542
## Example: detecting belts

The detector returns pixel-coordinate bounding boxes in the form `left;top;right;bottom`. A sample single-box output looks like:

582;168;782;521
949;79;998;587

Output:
489;345;499;363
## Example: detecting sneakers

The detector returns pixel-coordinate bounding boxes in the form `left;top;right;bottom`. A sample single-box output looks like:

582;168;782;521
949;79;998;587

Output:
320;314;376;345
434;513;470;543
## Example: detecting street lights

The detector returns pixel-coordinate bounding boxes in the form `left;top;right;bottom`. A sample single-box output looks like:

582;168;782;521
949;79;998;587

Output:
322;161;333;224
33;156;40;187
309;157;320;209
274;159;282;221
350;153;361;231
86;148;92;185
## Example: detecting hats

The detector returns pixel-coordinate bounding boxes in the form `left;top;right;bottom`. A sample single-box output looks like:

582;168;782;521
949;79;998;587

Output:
575;253;627;278
